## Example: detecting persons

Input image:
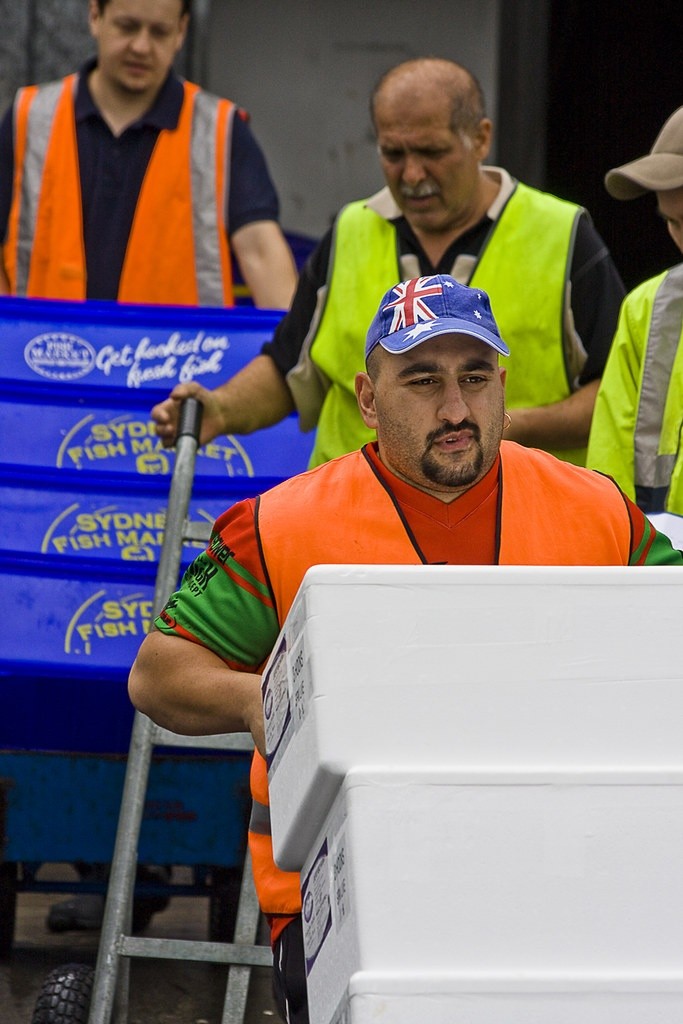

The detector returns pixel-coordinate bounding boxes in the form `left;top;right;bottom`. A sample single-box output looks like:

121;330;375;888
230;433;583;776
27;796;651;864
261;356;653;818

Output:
128;275;683;1024
150;57;630;471
0;0;299;935
586;102;683;552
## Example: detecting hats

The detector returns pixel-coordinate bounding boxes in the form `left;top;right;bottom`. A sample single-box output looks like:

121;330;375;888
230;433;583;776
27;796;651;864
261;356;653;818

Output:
365;275;510;362
605;105;683;200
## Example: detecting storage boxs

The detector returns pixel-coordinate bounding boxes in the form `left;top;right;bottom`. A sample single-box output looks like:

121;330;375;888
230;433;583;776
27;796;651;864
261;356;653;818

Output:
0;295;315;868
259;563;683;1024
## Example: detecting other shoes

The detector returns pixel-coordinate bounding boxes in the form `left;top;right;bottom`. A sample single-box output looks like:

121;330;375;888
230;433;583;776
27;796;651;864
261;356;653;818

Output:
47;891;152;934
